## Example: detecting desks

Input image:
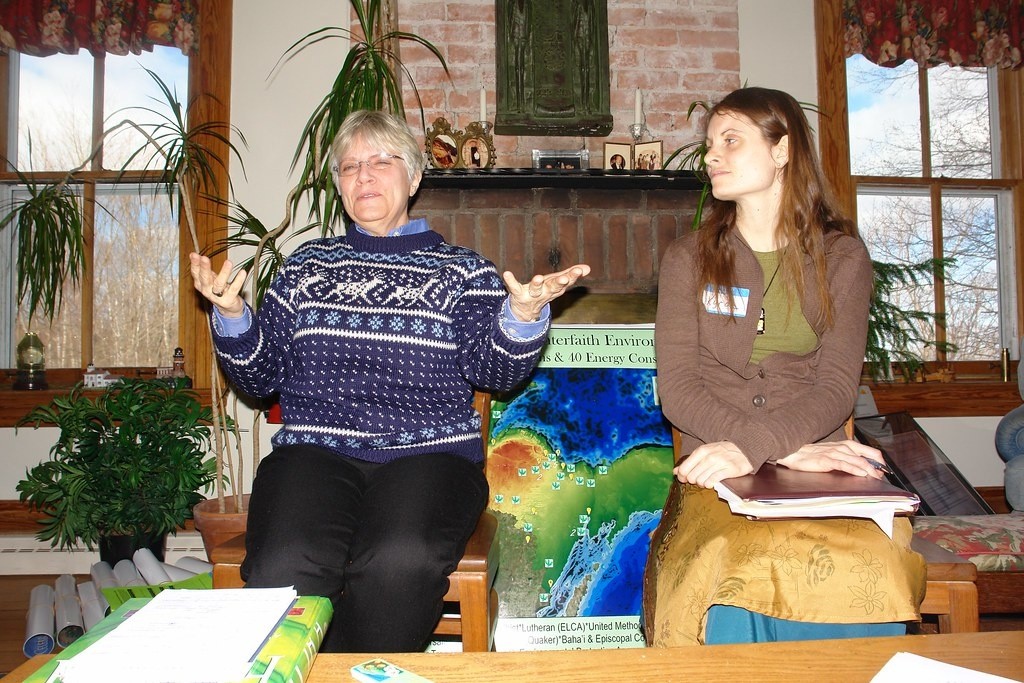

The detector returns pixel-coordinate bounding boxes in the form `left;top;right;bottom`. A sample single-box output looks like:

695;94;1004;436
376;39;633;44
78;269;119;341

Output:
0;630;1024;682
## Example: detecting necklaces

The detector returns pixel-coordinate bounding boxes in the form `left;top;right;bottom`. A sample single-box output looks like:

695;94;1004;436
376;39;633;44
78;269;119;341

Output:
763;223;802;299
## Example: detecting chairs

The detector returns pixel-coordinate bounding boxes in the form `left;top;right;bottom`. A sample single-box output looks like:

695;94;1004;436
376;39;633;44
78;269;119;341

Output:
211;390;501;651
672;408;979;635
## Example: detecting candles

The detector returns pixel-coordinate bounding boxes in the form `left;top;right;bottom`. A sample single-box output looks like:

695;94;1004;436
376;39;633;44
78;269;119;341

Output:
479;85;488;128
634;86;642;134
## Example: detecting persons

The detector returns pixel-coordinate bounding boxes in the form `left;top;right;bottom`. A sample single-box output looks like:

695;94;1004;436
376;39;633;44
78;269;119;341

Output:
611;155;623;169
639;88;925;643
638;154;656;170
187;109;593;653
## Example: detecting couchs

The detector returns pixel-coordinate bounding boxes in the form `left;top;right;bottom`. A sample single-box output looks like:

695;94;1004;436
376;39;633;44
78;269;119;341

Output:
994;336;1024;513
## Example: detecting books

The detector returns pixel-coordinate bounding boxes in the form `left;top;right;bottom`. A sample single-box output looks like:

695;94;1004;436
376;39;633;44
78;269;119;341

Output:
17;595;335;682
693;462;922;521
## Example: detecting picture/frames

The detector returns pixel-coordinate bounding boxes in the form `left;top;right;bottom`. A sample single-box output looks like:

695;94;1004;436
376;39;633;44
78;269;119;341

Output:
459;120;497;169
634;140;663;177
853;409;998;516
424;117;463;169
603;142;632;176
532;148;590;168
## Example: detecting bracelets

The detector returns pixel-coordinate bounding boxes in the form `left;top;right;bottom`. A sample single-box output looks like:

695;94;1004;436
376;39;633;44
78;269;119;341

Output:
529;316;540;324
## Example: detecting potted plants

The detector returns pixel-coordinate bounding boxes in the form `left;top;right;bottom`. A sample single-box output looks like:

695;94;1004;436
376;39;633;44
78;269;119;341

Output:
1;0;456;563
15;375;240;567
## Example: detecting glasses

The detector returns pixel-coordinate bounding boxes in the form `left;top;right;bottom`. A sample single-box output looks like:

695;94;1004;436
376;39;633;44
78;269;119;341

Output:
333;154;404;176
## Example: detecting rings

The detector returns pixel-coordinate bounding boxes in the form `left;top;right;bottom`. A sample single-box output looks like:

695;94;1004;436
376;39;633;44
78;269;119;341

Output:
212;288;223;297
